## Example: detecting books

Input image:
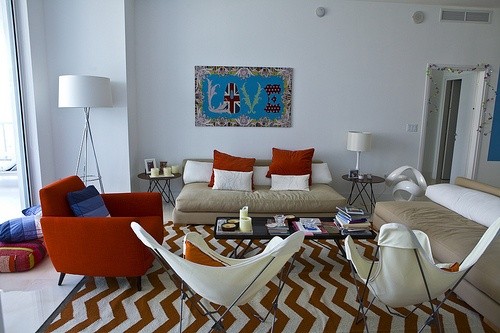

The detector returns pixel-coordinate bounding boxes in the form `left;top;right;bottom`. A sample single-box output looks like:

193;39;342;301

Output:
267;218;290;235
334;205;372;236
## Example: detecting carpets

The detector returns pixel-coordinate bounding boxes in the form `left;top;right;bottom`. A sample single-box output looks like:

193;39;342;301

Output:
35;220;497;333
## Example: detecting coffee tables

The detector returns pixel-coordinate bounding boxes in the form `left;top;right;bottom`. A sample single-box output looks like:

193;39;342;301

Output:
215;216;377;273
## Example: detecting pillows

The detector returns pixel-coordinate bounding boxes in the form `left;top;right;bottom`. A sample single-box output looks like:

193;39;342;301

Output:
212;168;253;192
0;184;111;272
208;150;256;190
270;173;310;191
265;148;315;186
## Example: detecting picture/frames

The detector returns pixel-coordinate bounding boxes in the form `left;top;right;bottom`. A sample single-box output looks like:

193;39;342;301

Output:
145;159;157;174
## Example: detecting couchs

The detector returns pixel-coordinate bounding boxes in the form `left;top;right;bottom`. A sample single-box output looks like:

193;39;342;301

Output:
374;176;500;333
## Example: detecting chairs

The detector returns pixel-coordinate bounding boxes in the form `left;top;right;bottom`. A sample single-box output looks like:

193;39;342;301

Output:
344;217;500;333
39;175;163;292
131;222;304;333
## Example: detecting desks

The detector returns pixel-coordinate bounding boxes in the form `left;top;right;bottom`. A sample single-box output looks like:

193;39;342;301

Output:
342;175;385;214
138;172;181;207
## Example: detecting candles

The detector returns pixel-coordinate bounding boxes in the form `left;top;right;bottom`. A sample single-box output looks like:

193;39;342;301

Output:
150;165;178;176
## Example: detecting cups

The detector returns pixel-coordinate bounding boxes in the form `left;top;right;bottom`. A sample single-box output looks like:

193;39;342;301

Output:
163;167;172;176
357;173;364;179
171;165;179;173
150;167;159;176
367;174;372;179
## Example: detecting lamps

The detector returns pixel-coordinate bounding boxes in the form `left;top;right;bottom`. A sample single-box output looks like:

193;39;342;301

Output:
58;75;112;193
347;131;372;176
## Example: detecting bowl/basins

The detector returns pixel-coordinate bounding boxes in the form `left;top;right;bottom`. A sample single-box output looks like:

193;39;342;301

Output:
221;222;236;231
226;219;239;228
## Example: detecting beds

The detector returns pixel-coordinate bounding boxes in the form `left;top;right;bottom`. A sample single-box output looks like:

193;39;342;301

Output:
172;158;346;224
